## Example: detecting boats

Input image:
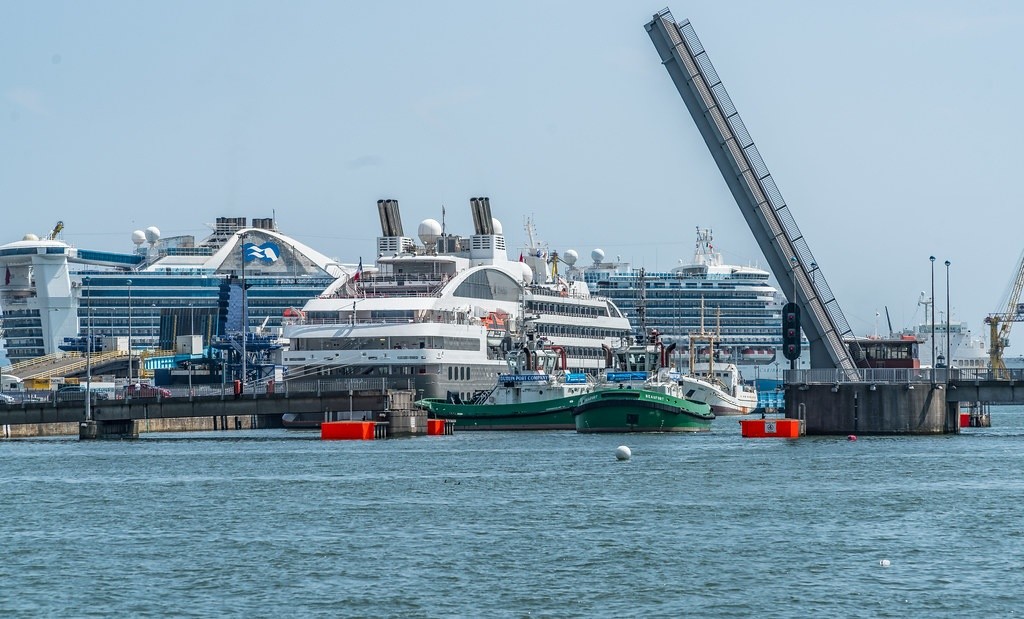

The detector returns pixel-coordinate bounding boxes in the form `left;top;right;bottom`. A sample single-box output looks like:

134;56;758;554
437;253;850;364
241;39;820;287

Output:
864;290;991;381
412;280;785;435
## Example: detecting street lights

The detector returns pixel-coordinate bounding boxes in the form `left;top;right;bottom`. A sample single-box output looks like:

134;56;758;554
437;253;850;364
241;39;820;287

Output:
85;276;93;420
236;232;251;385
944;260;951;380
125;279;133;385
929;256;936;383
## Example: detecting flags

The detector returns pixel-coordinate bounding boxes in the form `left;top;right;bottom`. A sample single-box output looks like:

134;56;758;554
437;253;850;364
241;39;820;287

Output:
4;264;11;286
354;261;363;281
519;253;523;262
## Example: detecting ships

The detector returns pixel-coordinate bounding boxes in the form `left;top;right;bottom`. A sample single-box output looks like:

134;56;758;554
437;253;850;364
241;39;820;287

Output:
281;198;637;402
578;226;814;380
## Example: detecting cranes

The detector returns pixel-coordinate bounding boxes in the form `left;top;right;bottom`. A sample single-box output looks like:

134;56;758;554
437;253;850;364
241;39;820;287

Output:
984;248;1024;381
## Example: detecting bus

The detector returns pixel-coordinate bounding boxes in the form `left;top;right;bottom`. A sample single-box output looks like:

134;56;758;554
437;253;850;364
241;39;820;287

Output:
844;334;928;369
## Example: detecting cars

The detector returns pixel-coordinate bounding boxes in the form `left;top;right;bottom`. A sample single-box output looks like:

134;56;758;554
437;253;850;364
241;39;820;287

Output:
48;386;109;401
121;384;173;399
0;393;16;405
